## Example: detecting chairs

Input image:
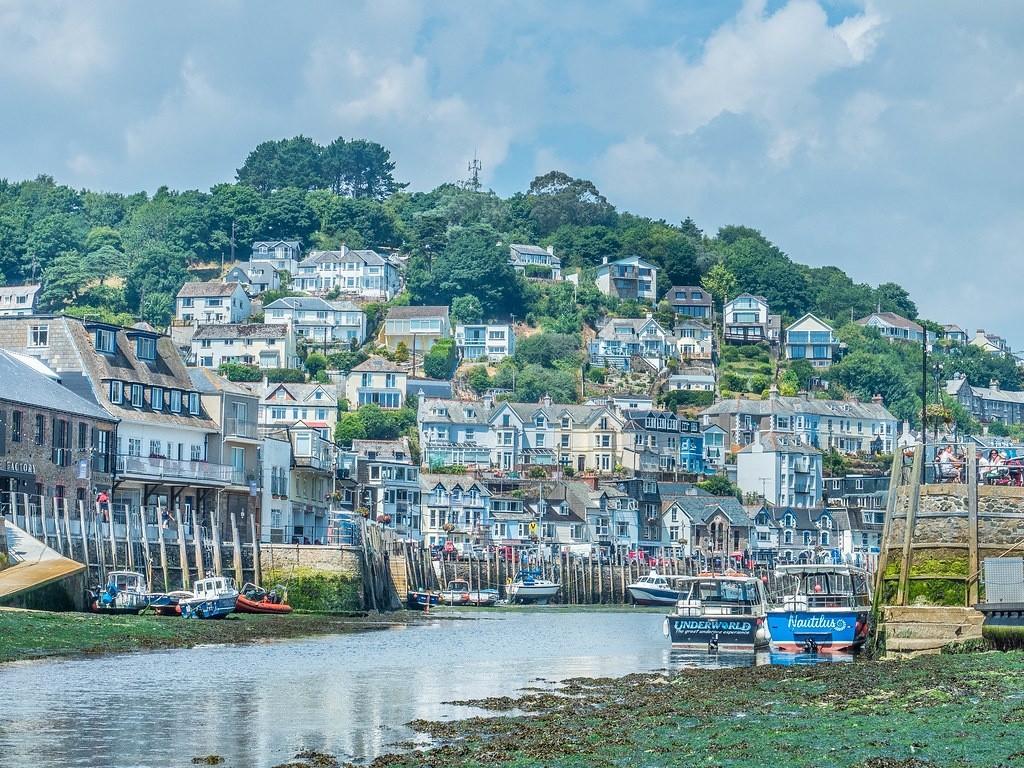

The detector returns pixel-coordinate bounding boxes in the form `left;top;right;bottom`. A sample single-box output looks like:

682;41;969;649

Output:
932;461;938;483
936;460;957;483
987;461;1006;485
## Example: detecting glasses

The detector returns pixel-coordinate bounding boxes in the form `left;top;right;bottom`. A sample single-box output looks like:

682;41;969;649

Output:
991;453;997;454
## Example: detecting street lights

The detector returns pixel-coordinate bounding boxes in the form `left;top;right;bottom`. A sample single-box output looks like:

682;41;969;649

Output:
933;362;944;460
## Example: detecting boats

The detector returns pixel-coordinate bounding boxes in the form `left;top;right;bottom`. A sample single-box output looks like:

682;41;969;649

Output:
235;582;293;614
93;571;148;615
764;564;875;653
439;578;470;605
408;588;441;607
626;567;690;606
665;532;771;654
143;590;195;615
469;589;499;607
180;567;238;620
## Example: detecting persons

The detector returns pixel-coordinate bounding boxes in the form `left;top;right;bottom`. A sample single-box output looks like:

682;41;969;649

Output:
96;491;112;521
161;508;172;527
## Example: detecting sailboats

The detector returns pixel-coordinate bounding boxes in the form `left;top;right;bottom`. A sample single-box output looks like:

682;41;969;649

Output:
504;483;561;604
700;506;754;600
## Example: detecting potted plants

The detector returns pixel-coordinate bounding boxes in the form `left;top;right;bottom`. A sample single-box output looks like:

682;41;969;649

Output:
905;451;914;464
919;404;953;425
